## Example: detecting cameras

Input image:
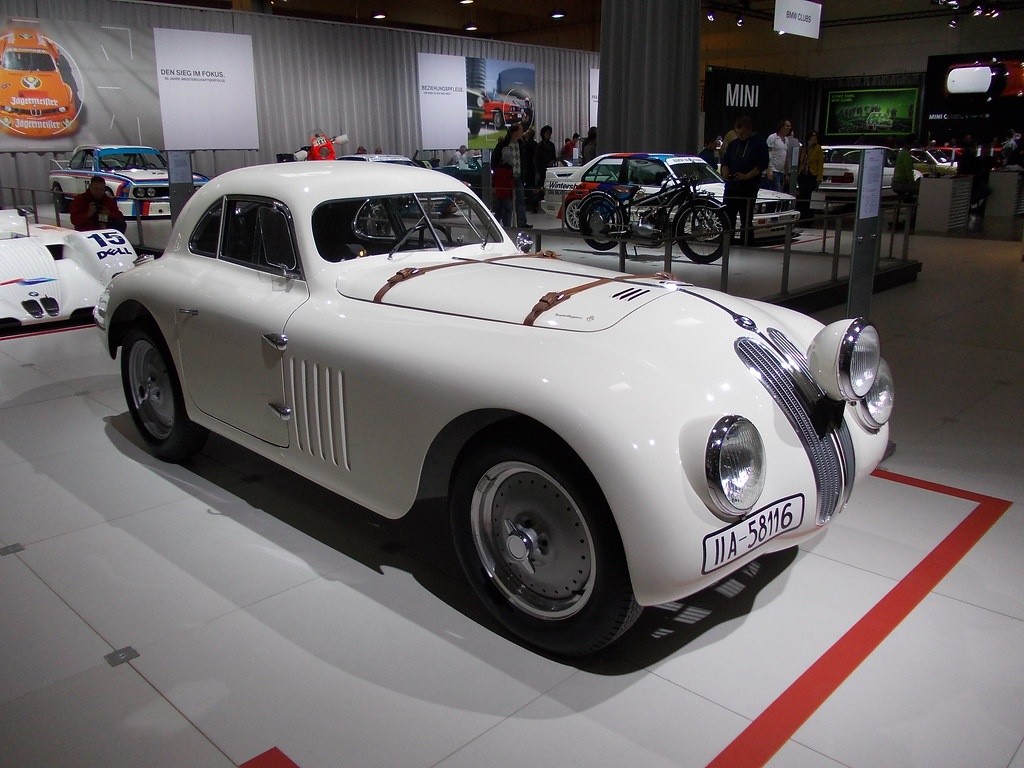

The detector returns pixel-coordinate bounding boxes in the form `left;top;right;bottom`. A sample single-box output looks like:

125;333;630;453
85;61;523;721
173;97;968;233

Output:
92;200;103;210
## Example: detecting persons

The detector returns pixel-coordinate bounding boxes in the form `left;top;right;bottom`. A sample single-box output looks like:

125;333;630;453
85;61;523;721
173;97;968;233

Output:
70;177;127;235
891;128;1024;226
354;146;383;155
700;117;824;246
433;123;596;230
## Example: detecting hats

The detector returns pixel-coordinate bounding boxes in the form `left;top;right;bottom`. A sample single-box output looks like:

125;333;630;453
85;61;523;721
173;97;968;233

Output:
572;133;580;138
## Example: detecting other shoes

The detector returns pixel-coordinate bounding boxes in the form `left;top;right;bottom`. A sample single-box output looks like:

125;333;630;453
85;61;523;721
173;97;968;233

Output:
518;224;533;229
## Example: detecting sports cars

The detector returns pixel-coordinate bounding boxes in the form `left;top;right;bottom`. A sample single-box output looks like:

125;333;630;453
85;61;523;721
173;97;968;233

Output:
0;28;81;141
324;155;473;219
90;158;897;661
0;205;140;330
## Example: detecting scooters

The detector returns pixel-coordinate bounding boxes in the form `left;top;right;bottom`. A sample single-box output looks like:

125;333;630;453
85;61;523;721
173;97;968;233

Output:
576;171;736;264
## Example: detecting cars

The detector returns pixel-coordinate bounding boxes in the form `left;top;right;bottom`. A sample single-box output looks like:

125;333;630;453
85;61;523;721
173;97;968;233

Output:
47;145;211;219
820;142;1006;204
538;151;805;244
464;87;531;136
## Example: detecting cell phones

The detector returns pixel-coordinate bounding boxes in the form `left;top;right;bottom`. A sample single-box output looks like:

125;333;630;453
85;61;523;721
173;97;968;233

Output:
728;175;736;178
